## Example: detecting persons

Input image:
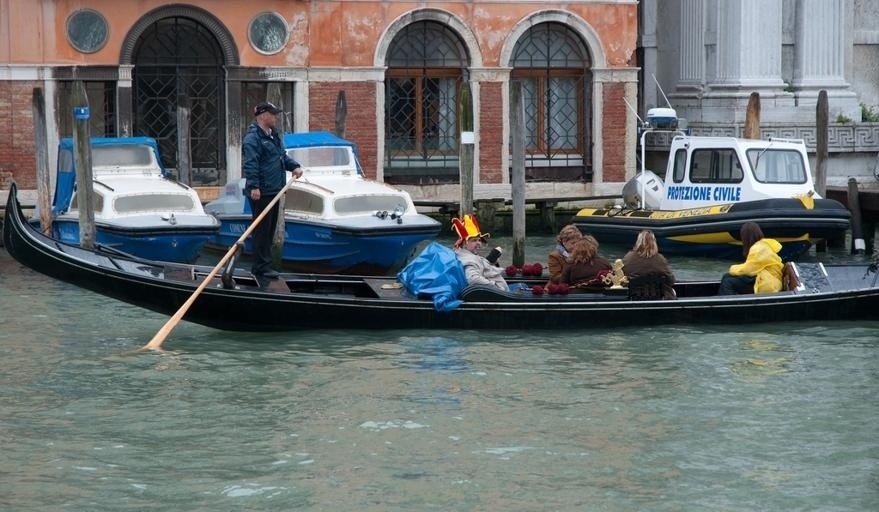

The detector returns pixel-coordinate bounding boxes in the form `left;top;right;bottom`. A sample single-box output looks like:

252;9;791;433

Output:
543;225;613;294
622;229;678;300
454;235;510;293
242;102;303;278
718;222;785;295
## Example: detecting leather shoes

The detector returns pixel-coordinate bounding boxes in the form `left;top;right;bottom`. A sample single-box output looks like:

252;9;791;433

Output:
250;270;280;279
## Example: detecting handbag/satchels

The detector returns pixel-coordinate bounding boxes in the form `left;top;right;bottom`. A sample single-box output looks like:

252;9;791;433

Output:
781;261;806;291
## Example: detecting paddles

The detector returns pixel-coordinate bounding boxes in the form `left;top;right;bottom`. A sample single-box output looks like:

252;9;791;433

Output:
144;175;298;350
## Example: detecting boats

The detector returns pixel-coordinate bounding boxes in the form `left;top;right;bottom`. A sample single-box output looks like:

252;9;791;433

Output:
201;132;445;271
566;105;856;264
2;179;878;336
25;129;224;266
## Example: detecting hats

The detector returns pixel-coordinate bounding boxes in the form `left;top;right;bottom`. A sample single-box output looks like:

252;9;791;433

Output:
254;102;282;116
450;213;490;247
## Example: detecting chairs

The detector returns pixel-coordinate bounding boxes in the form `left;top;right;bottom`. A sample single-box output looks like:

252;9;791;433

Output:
629;272;662;299
